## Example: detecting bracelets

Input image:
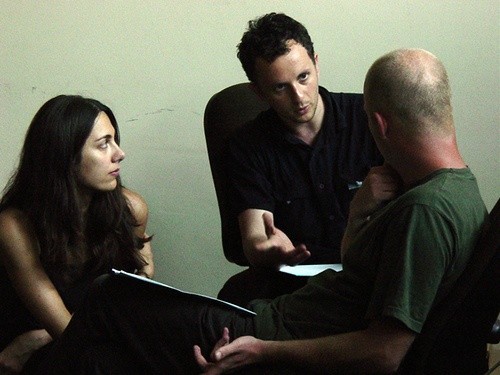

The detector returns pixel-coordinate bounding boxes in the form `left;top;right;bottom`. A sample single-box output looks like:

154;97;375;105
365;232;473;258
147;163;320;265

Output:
136;269;153;280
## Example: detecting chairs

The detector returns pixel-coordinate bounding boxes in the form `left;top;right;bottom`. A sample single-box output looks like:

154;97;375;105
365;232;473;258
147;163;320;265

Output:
395;198;500;375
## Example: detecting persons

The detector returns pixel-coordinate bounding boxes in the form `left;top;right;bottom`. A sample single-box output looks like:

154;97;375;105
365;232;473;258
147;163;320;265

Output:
193;48;489;375
0;93;155;374
218;12;384;308
